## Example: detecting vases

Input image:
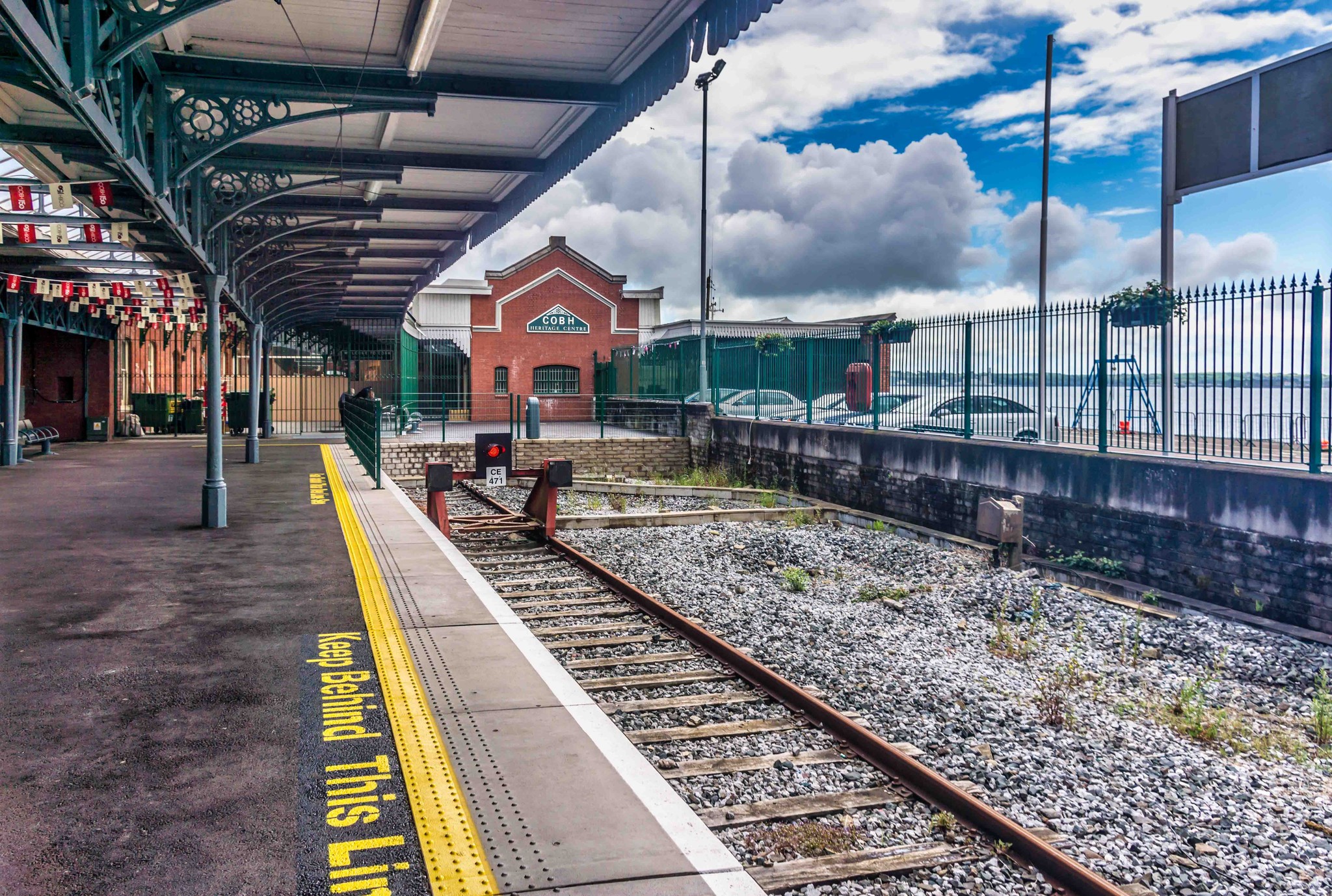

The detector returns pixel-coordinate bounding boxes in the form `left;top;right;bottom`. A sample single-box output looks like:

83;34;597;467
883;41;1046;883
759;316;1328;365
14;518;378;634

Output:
1112;309;1170;327
762;344;785;354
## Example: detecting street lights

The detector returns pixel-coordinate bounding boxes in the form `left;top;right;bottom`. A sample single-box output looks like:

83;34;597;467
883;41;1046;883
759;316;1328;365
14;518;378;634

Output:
693;57;727;404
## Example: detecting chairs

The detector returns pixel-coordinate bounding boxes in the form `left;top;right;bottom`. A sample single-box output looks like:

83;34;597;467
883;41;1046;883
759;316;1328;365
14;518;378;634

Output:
382;405;398;431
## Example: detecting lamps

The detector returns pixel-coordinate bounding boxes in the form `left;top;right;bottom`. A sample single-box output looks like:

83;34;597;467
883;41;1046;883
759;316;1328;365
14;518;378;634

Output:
396;0;452;78
363;181;382;201
0;142;61;184
345;247;357;256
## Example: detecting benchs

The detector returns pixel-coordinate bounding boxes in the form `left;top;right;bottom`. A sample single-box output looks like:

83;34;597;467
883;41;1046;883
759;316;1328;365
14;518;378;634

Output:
0;419;59;462
404;407;423;434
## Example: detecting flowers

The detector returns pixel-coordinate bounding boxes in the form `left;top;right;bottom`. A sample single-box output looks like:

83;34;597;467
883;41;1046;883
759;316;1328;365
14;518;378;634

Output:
1093;280;1188;328
755;332;795;356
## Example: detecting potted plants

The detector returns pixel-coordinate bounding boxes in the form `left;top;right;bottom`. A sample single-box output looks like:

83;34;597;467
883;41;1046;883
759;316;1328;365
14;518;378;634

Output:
867;319;918;343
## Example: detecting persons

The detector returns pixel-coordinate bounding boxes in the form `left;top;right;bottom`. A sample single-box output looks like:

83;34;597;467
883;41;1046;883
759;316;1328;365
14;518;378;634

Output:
339;388;356;427
364;387;381;419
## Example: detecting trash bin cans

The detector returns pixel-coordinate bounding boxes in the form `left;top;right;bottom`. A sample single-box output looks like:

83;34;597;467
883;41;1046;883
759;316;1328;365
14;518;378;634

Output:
131;392;185;434
224;388;276;430
525;397;539;439
181;398;204;434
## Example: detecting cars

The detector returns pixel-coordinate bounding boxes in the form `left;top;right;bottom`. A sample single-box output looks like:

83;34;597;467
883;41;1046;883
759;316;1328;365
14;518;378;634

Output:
769;392;926;426
715;390;806;417
685;388;746;405
843;390;1060;443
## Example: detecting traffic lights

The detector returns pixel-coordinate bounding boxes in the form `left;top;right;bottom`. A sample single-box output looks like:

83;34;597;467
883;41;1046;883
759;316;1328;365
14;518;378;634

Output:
476;432;513;479
